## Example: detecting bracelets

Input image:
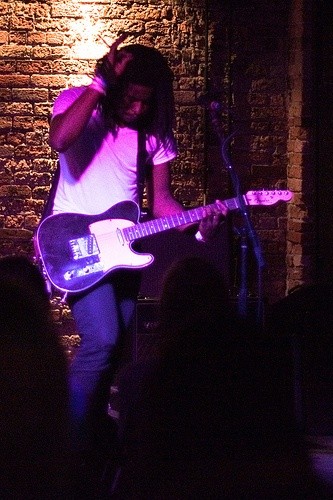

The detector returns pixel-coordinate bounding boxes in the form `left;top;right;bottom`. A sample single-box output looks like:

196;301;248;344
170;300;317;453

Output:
194;231;209;246
90;58;117;96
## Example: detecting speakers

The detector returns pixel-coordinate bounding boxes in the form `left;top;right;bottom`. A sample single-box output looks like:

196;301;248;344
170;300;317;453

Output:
136;298;265;391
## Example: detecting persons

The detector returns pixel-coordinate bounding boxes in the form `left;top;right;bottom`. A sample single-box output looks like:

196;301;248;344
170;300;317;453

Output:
0;255;95;500
106;257;312;500
34;33;230;456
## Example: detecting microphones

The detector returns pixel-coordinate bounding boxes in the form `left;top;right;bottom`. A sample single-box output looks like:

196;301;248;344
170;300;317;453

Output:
207;101;229;142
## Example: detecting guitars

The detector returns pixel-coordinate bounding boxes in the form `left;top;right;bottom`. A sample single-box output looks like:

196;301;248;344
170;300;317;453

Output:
35;189;293;296
